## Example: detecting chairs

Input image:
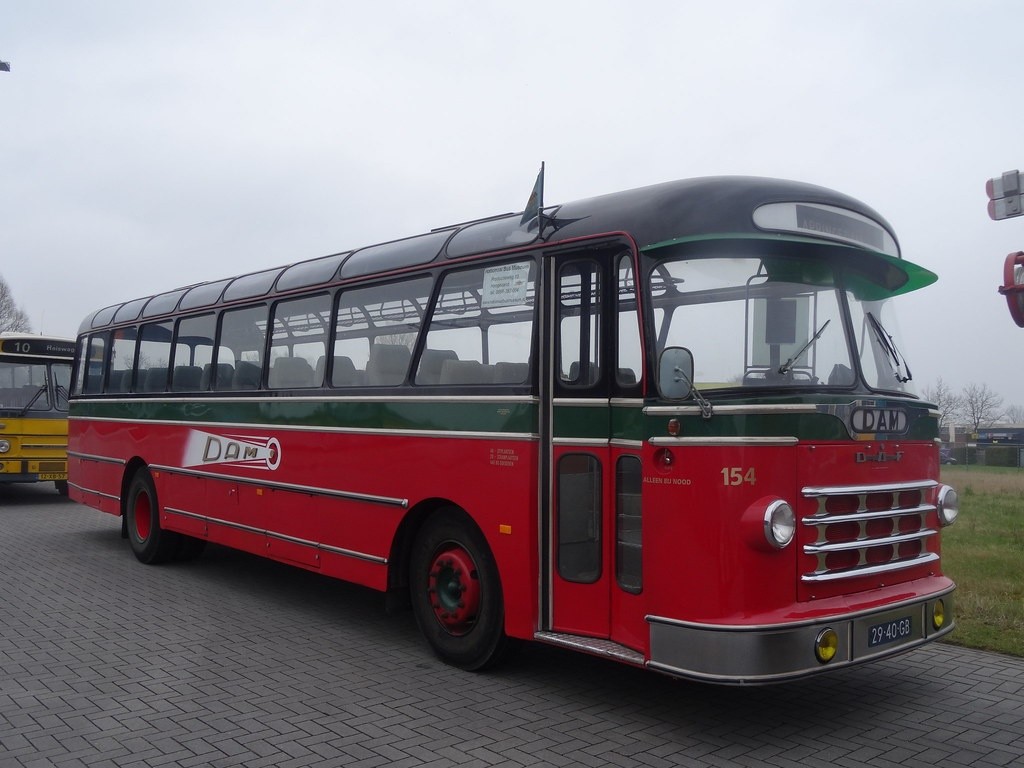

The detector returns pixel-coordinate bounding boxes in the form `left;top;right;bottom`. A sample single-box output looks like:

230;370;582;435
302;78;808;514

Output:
0;383;61;409
84;342;637;386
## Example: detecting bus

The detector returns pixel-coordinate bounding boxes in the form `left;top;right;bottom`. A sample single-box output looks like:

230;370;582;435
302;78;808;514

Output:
0;328;116;503
62;160;961;690
